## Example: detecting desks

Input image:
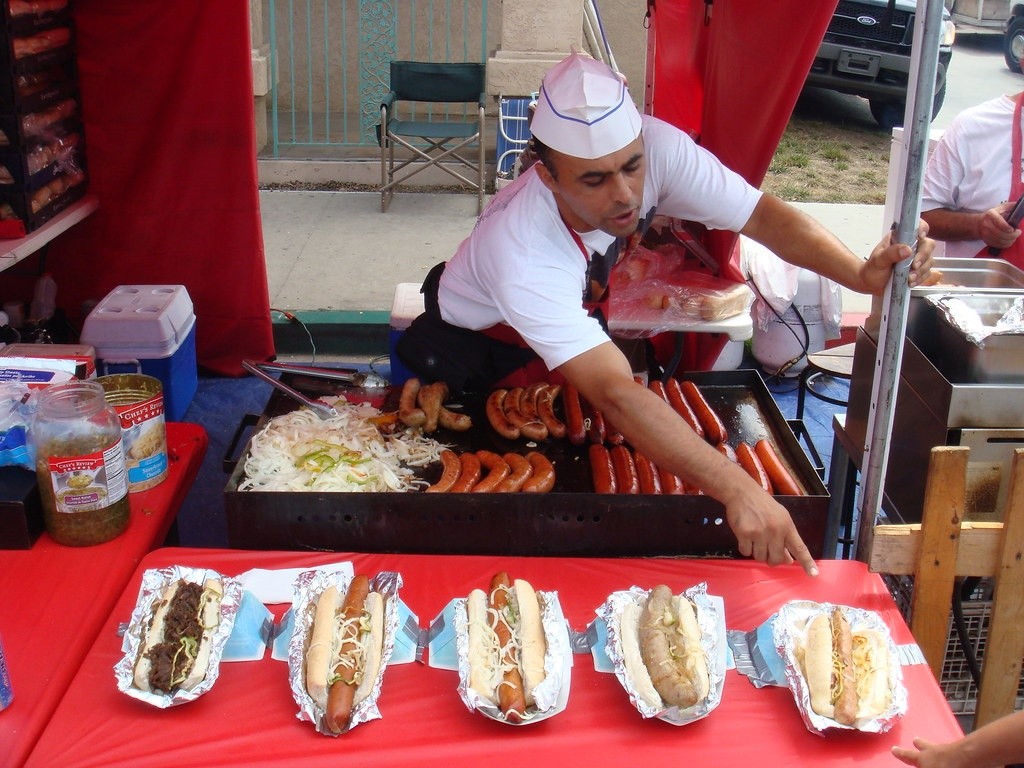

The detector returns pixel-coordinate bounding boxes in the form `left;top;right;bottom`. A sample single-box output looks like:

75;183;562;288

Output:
17;543;967;768
0;415;210;768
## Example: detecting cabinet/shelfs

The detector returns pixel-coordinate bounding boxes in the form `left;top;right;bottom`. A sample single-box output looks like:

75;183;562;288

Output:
0;0;91;233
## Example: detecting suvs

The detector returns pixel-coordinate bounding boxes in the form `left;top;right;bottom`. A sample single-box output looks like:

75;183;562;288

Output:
806;0;956;132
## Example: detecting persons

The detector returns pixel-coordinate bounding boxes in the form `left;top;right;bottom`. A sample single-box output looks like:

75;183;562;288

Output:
388;45;946;576
891;709;1024;768
920;91;1024;291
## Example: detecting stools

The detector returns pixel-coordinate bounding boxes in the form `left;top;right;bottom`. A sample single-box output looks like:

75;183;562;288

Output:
792;342;858;421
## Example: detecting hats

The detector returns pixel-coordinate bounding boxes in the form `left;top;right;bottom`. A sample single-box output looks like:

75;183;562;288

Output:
530;52;643;161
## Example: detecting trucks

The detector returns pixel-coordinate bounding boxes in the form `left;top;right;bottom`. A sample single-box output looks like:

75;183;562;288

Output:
942;0;1024;75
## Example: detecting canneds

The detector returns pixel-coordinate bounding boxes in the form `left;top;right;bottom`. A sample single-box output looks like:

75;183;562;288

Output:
32;379;130;546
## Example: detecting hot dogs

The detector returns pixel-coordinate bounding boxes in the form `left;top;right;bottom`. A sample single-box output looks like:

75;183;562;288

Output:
134;579;222;695
307;575;384;735
466;571;546;724
804;608;888;728
620;584;709;710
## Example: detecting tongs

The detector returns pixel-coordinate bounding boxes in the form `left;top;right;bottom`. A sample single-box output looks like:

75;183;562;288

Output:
240;358;391;424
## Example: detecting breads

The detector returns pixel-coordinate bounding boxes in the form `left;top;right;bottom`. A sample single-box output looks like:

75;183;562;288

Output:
619;254;752;322
7;0;85;213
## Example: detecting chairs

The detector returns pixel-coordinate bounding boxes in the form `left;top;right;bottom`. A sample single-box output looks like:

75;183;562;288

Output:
372;56;490;222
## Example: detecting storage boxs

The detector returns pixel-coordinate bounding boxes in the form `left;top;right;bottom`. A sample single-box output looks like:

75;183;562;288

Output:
81;286;200;422
385;281;426;387
924;292;1024;383
863;254;1024;330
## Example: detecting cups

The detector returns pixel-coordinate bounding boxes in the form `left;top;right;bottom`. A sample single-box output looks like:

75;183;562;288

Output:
90;373;170;494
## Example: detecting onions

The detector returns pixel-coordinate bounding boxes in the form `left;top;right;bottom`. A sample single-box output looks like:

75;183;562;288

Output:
235;393;455;494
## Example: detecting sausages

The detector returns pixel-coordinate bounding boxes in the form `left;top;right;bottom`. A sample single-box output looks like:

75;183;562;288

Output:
396;379;803;495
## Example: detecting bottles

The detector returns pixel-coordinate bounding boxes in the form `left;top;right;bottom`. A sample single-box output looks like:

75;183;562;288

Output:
33;380;130;547
